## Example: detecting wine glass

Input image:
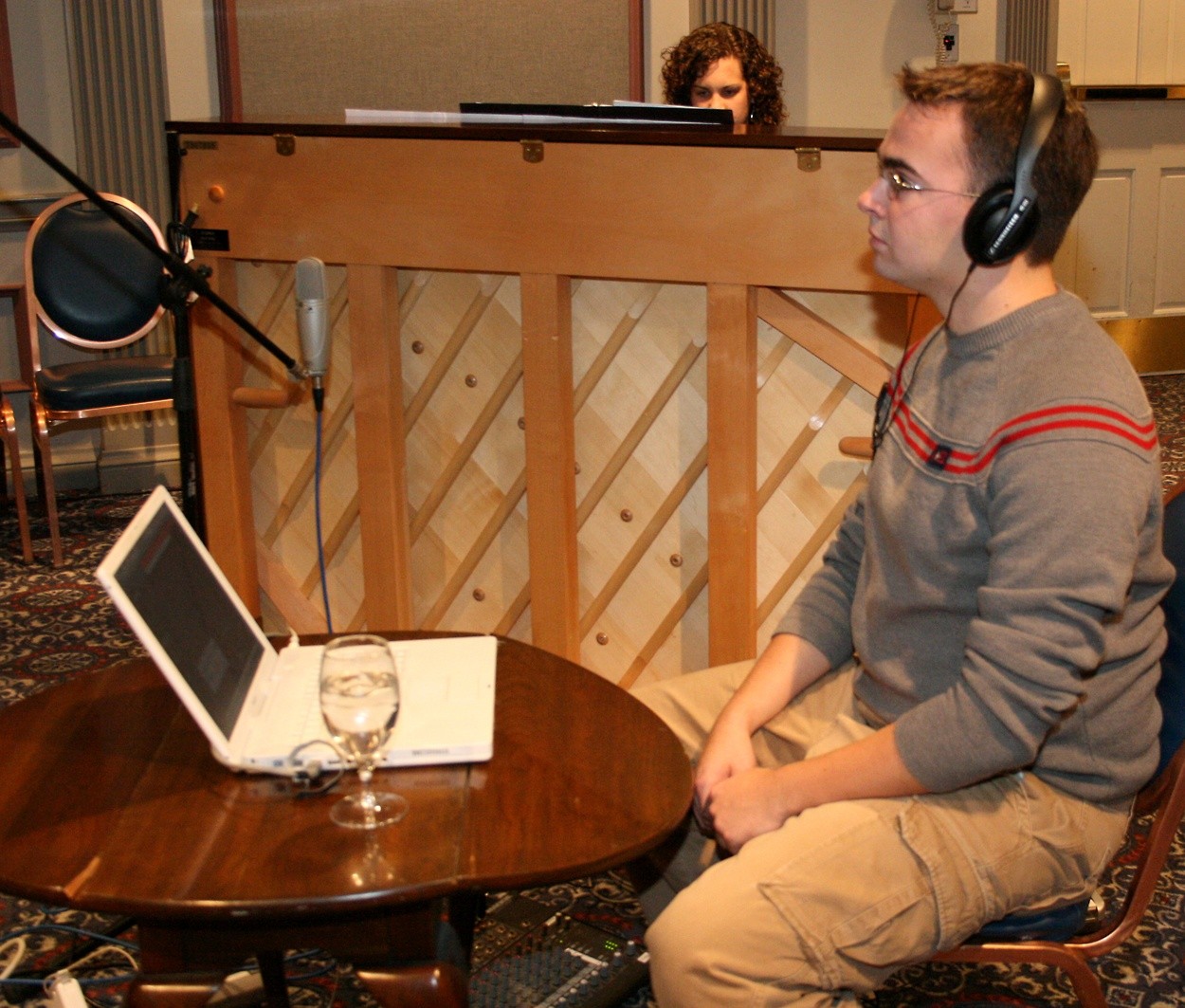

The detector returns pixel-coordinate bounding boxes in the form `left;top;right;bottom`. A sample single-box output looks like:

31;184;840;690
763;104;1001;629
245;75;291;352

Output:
319;634;408;828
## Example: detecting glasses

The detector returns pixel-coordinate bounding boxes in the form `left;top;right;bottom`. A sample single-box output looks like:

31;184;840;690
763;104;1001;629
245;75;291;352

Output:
876;157;979;201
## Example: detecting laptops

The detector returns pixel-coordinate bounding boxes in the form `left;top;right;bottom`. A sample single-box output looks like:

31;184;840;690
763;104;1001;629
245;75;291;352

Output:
95;486;499;773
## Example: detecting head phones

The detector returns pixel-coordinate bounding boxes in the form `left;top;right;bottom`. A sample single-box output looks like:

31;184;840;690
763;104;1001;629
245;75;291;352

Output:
961;69;1067;269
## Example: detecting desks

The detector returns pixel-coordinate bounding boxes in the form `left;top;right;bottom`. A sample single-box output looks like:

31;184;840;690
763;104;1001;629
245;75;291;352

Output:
0;628;693;1008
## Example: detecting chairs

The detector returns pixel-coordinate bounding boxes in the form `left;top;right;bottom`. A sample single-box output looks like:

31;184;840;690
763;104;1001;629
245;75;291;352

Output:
25;195;176;565
850;490;1185;1008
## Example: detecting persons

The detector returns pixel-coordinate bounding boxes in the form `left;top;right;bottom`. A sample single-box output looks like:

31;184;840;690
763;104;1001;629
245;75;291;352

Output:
659;22;787;123
626;63;1175;1008
